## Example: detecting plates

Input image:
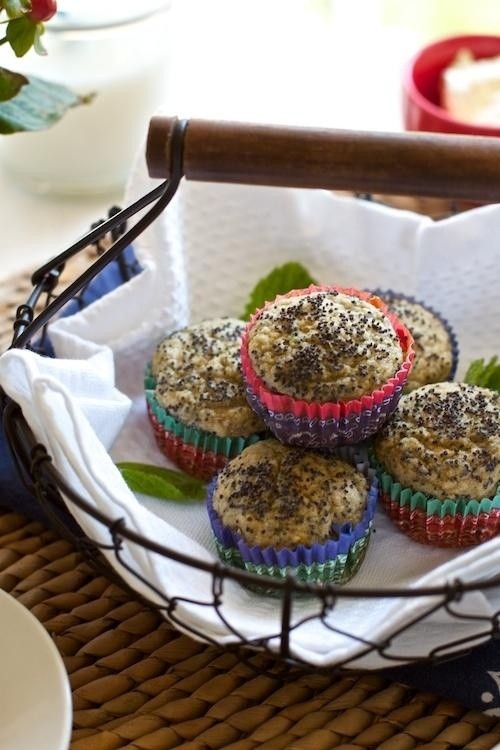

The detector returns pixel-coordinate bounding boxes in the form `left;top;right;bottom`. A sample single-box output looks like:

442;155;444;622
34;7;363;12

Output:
0;589;74;750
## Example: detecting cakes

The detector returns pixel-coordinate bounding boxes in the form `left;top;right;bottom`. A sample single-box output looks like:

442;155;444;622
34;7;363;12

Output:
364;288;460;395
143;317;272;481
206;439;380;600
239;283;416;449
374;381;500;549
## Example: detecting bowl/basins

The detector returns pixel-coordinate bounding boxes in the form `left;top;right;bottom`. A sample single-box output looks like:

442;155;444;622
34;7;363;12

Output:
399;36;499;139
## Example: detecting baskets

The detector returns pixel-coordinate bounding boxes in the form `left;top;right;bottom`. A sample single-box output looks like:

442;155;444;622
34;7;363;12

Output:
5;161;499;678
